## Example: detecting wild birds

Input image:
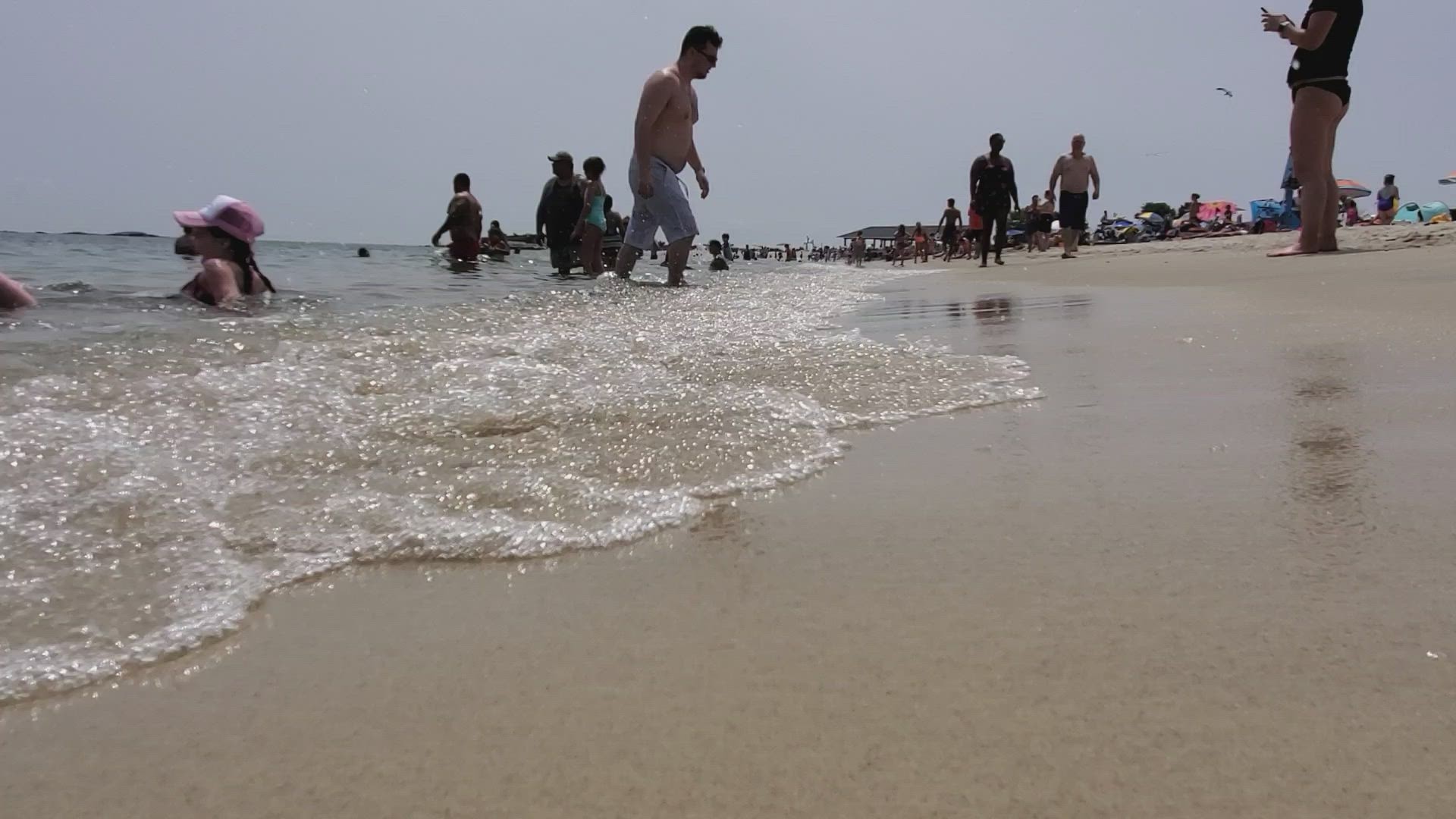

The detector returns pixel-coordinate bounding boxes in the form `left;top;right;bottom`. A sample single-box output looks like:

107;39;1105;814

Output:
1215;88;1233;97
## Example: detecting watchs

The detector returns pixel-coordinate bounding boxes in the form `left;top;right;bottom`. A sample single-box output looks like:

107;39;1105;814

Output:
695;167;705;173
1278;21;1290;32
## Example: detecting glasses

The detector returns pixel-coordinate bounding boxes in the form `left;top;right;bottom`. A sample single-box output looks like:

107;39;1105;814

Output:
694;46;716;65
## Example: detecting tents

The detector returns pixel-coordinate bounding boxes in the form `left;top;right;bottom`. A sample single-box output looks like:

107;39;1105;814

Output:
1390;200;1452;223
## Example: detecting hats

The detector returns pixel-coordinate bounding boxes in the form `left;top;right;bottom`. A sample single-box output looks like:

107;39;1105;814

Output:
547;151;573;163
174;195;264;243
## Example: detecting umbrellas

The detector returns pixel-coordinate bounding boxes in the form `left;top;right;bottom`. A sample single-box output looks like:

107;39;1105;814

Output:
1438;170;1456;184
1137;212;1164;225
1336;178;1371;199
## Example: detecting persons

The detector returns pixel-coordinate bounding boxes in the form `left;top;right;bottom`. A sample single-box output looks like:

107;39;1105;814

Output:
0;272;36;310
889;198;983;266
708;230;867;270
1184;175;1400;226
1260;0;1364;257
431;173;511;262
1024;190;1056;253
173;195;277;309
968;134;1022;268
358;248;372;258
616;25;723;286
536;151;658;278
1049;133;1100;259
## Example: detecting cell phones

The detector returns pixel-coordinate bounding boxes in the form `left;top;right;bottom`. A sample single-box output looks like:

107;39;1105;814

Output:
1261;7;1267;13
